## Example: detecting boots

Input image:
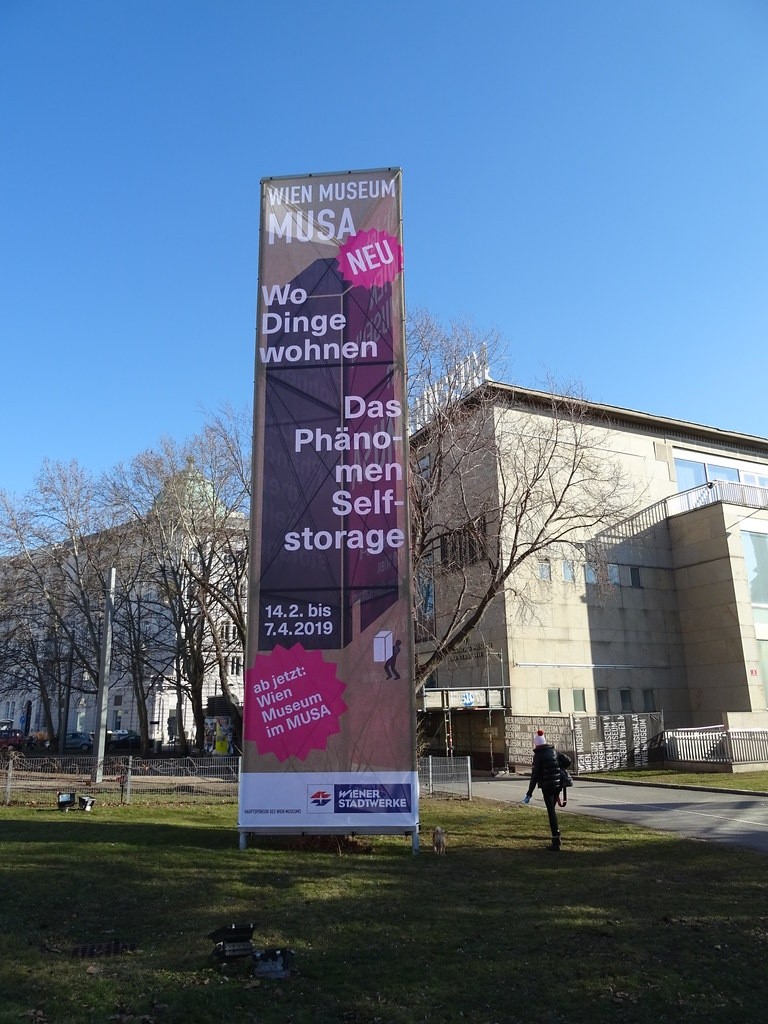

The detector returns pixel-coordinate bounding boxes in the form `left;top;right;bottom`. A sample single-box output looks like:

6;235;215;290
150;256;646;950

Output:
546;832;562;852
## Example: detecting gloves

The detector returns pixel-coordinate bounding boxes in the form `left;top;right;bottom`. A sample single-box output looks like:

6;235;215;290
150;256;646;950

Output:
527;791;532;797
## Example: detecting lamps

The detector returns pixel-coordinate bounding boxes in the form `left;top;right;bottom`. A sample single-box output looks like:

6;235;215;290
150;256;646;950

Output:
78;796;97;811
208;923;256;957
57;792;75;813
250;948;296;980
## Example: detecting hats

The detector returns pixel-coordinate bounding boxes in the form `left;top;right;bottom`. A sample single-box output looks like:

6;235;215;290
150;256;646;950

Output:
534;730;546;745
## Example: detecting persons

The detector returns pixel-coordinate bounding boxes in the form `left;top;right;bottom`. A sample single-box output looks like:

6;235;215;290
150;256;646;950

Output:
523;730;573;851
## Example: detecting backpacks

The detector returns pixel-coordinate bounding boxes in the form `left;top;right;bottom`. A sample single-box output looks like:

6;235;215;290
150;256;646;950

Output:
557;751;573;787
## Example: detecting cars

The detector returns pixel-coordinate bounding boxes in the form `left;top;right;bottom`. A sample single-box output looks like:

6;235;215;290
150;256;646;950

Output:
46;732;94;752
106;735;155;752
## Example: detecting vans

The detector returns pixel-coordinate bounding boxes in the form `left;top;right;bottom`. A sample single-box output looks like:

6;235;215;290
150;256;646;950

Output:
0;729;25;751
